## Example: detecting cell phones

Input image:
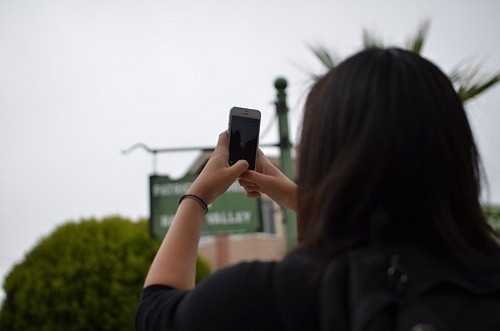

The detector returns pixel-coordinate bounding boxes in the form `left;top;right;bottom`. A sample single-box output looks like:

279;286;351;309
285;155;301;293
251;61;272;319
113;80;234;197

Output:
228;107;261;179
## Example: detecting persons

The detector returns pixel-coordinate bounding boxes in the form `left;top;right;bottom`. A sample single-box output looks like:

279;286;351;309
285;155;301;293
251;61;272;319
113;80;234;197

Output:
136;47;500;330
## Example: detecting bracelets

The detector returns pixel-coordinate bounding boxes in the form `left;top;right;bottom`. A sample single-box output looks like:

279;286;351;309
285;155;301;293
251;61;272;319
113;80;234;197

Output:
180;194;208;215
